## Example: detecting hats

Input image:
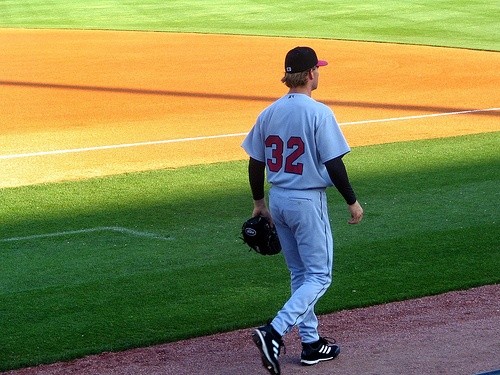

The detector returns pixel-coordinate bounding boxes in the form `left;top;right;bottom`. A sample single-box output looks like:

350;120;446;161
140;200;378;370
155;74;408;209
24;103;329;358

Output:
285;47;328;74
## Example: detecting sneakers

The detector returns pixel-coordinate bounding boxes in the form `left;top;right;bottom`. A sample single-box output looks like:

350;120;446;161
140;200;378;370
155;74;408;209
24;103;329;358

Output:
252;325;286;375
300;337;340;365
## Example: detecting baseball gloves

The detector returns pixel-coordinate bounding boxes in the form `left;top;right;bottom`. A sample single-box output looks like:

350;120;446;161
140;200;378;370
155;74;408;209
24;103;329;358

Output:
237;214;282;256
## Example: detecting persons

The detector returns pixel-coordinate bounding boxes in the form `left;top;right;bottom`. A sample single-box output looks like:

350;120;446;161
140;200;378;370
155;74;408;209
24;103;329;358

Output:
241;47;364;375
